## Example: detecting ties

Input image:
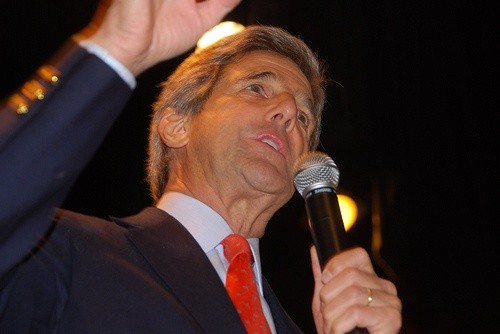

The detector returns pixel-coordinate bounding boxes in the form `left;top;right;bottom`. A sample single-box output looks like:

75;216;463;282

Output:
220;234;272;334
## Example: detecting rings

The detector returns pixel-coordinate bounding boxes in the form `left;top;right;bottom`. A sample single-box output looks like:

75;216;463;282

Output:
367;288;372;306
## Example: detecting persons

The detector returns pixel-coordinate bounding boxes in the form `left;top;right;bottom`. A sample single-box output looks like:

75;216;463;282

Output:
0;0;402;334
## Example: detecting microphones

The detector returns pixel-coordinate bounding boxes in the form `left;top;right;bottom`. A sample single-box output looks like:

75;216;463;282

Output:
292;150;351;274
